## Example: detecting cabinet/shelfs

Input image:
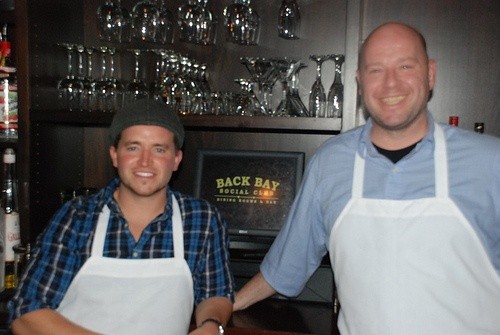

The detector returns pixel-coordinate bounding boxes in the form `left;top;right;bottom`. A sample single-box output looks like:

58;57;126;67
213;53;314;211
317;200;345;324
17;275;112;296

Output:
0;0;363;308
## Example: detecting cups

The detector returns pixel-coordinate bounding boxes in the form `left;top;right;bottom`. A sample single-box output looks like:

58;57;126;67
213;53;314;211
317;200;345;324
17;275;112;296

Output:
206;88;252;117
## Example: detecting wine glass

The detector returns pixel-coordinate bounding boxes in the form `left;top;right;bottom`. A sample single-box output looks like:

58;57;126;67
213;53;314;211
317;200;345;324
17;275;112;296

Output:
176;0;217;46
130;0;176;44
222;0;262;45
55;40;212;115
95;0;130;42
278;0;302;40
234;56;308;118
308;54;331;118
327;55;343;118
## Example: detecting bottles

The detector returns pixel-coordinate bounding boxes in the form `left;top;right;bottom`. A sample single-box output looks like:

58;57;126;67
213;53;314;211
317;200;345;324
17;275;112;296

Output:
0;148;21;291
448;116;458;128
474;122;485;134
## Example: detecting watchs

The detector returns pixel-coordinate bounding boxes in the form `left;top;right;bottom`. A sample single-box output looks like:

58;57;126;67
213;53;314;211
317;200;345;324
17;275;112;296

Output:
202;319;224;335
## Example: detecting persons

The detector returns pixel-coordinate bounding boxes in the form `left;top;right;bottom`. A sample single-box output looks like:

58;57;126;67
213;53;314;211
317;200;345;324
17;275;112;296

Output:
230;22;500;335
5;98;235;335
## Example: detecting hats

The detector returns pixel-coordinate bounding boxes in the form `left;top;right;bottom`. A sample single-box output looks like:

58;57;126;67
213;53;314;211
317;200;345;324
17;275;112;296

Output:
110;100;183;151
